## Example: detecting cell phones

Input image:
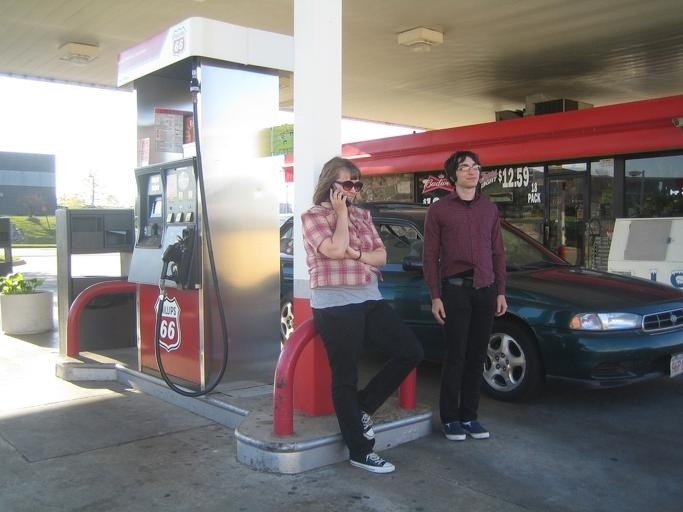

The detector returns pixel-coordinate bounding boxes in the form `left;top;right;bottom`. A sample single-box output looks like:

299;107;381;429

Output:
330;184;352;207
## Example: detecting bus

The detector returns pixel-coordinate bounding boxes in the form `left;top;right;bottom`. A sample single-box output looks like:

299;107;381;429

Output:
341;95;683;288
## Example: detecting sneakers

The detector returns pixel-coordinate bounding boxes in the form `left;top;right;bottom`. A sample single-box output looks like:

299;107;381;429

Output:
443;421;466;440
349;450;396;473
463;420;489;439
360;409;375;440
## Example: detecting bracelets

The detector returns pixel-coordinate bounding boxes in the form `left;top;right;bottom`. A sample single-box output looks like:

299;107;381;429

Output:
356;249;362;260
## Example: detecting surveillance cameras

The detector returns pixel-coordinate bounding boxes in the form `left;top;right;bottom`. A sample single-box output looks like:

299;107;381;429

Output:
672;118;683;127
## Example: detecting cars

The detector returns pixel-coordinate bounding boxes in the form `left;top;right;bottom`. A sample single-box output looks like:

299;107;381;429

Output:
280;202;683;404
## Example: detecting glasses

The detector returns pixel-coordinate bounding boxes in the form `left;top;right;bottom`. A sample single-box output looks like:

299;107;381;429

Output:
459;164;481;172
332;180;363;192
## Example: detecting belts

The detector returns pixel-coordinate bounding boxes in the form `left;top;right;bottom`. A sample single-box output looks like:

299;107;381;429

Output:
448;276;473;288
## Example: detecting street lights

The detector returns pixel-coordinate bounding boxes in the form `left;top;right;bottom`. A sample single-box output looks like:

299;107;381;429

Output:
629;168;646;214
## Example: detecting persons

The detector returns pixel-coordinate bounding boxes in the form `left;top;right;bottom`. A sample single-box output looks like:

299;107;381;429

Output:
423;151;508;441
299;156;415;474
